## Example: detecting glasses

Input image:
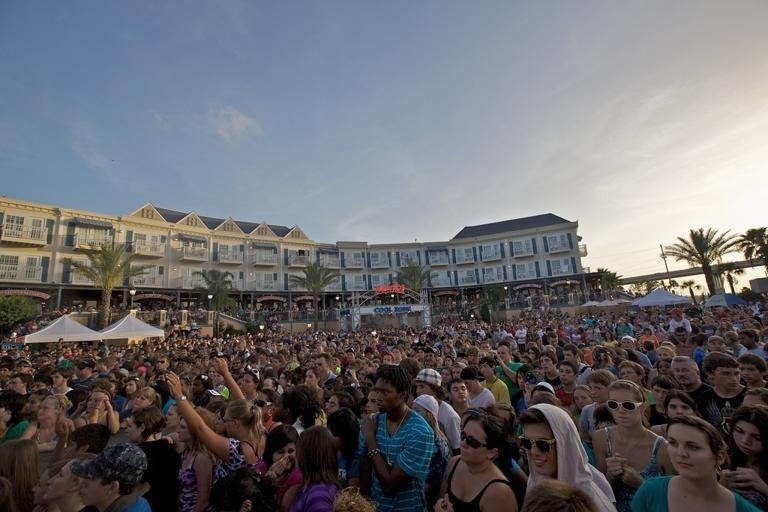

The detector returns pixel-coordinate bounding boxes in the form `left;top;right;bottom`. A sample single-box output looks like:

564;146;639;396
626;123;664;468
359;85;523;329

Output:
250;370;260;379
312;362;321;367
517;434;556;452
459;431;489;449
606;399;643;411
251;399;272;409
3;316;602;361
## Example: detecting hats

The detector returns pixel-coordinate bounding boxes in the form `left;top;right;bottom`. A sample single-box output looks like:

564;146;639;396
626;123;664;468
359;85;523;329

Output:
207;384;230;401
531;381;555;397
415;368;442;389
75;423;110;453
460;366;485;383
71;440;148;488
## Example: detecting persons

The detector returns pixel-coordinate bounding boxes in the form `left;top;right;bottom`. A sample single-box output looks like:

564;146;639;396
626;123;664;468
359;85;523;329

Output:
1;285;767;511
